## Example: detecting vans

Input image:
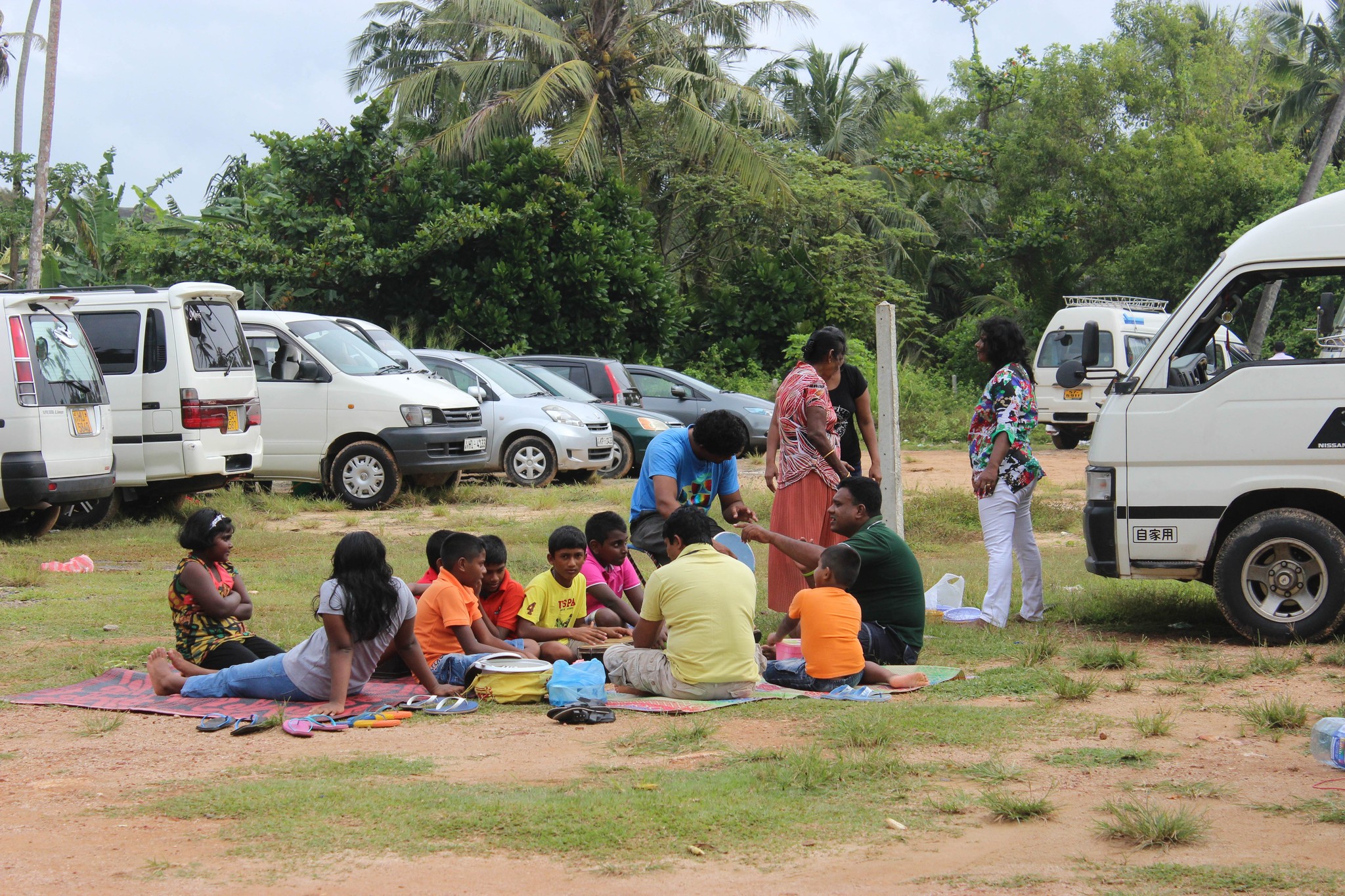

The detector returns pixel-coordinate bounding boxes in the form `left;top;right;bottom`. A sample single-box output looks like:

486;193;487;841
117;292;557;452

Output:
0;281;487;540
1030;295;1261;450
1056;190;1345;648
492;355;644;410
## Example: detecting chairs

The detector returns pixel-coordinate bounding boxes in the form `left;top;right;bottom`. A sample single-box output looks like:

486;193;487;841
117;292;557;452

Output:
271;344;306;381
244;345;277;380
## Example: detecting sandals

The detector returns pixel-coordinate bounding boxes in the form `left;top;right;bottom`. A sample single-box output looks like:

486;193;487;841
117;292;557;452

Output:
545;697;607;718
552;707;616;723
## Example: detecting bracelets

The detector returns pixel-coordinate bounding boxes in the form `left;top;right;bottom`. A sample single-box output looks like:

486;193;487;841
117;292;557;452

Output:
823;450;834;458
846;472;850;477
802;570;816;576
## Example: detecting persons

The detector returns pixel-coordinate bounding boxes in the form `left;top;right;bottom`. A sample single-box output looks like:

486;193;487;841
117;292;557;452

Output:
968;316;1044;629
168;507;289;678
603;505;768;700
514;526;633;668
147;531;466;717
397;530;541;698
733;477;926;665
629;409;762;645
764;326;882;639
579;511;668;650
762;545;930;692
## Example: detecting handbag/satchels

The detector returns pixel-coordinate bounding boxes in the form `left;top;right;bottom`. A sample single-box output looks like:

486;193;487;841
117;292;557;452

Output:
924;572;965;612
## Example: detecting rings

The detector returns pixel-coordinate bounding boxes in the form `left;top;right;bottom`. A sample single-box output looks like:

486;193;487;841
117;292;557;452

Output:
986;484;991;489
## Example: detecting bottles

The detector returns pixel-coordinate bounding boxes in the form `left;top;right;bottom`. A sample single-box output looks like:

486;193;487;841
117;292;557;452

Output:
1309;718;1345;770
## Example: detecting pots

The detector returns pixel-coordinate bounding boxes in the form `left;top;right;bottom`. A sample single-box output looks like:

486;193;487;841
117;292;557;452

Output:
474;659;553;675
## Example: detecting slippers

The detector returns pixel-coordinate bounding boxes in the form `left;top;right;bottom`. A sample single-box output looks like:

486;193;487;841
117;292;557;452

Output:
282;717;313;737
331;712;400;728
820;684;857;700
303;715;348;730
843;685;892;702
399;694;446;709
196;713;236;732
426;697;479;715
345;704;412;721
230;714;268;735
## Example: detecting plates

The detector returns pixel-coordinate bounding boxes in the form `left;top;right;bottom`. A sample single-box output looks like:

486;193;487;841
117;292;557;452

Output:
713;532;755;574
937;604;982;623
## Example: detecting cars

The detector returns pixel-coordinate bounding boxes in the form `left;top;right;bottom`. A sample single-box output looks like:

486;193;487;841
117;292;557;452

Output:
624;363;776;452
408;349;686;490
